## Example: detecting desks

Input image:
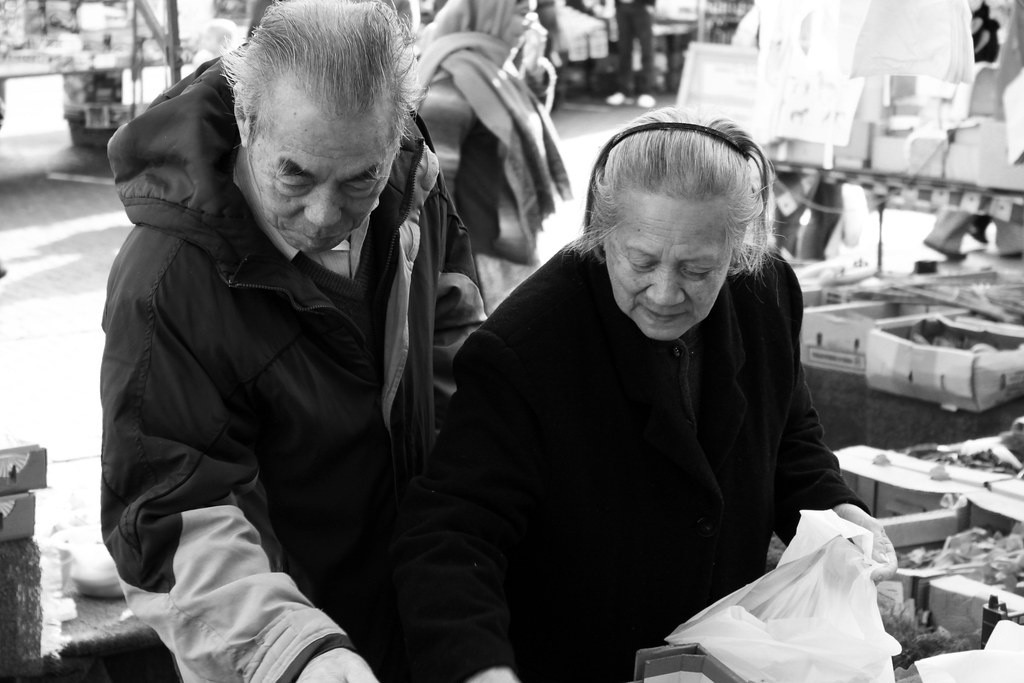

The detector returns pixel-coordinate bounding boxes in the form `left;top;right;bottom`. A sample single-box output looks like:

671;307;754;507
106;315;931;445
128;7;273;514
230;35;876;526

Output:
0;60;172;188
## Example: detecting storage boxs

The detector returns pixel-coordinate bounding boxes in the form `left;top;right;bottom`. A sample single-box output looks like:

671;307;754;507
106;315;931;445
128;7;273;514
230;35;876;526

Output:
632;62;1024;682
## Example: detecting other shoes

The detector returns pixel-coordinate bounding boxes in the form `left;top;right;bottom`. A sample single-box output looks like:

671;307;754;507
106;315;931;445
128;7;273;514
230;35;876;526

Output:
636;94;656;108
605;93;633;106
924;238;967;261
968;219;990;246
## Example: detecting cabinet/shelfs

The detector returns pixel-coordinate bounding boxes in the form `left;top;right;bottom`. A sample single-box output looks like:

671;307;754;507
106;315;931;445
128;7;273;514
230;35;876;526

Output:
764;157;1024;412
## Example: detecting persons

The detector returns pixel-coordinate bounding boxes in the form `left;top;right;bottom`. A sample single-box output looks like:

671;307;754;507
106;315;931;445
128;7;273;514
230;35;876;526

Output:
603;0;658;107
926;204;997;260
421;109;896;683
111;0;484;683
412;0;556;320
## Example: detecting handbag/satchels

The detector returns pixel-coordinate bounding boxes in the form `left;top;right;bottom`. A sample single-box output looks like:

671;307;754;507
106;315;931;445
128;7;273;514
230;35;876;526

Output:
663;509;902;683
